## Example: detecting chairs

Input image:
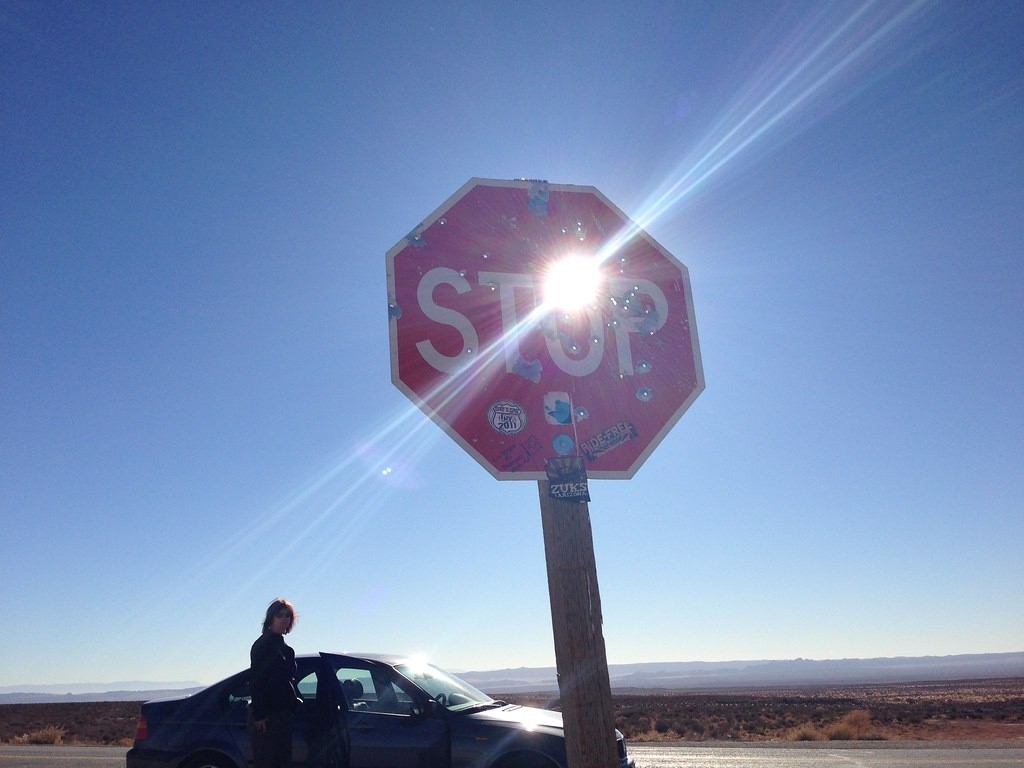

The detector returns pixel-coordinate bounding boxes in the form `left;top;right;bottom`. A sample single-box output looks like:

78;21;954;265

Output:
344;678;370;711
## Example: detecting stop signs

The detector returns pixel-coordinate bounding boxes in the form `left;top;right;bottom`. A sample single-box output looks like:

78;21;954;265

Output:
385;178;706;480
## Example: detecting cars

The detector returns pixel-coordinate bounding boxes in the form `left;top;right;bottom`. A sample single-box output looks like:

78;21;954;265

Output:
125;651;637;768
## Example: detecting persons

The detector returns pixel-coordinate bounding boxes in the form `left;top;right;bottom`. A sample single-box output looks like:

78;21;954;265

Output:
250;600;298;768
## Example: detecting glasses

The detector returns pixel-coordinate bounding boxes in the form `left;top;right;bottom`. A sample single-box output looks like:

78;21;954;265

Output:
274;611;292;618
